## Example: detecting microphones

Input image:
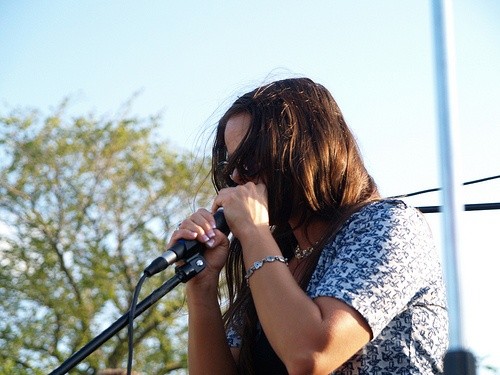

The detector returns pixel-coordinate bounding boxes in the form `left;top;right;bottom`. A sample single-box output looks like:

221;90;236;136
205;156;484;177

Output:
142;205;234;278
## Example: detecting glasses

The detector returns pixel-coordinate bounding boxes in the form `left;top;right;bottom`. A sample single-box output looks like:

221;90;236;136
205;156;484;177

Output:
217;152;263;181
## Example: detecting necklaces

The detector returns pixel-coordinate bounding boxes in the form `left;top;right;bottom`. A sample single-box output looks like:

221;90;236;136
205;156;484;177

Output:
294;233;328;260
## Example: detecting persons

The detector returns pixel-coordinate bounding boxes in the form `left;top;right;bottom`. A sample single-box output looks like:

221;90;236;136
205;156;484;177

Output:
167;76;452;375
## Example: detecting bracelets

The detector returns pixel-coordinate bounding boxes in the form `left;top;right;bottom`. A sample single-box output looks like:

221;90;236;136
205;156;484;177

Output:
246;255;289;289
